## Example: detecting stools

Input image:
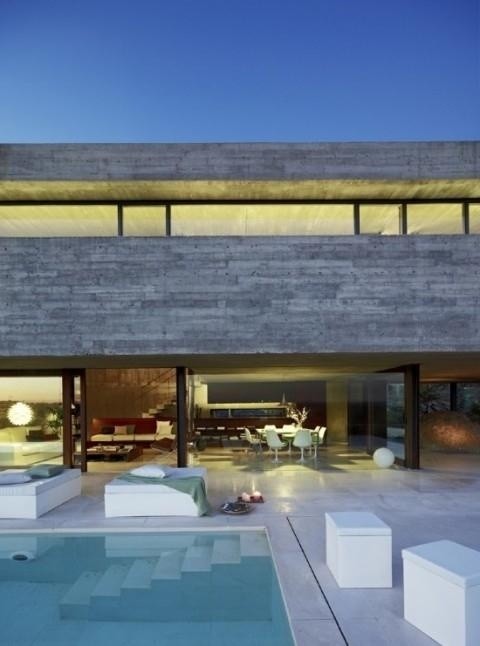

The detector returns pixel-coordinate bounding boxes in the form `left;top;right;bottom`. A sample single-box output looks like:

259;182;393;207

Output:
322;510;479;646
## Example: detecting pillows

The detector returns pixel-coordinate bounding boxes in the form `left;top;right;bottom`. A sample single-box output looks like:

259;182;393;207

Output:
114;424;135;436
153;420;174;435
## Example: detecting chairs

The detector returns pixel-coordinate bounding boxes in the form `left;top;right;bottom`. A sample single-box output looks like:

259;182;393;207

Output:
242;420;327;466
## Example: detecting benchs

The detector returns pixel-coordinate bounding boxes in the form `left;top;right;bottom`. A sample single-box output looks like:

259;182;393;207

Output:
88;417;177;452
1;463;209;520
